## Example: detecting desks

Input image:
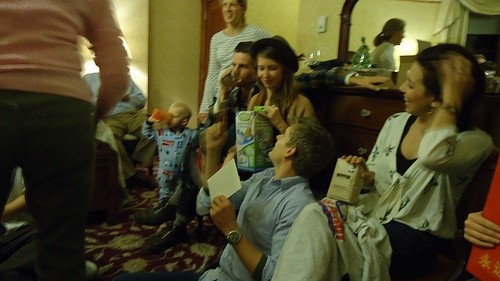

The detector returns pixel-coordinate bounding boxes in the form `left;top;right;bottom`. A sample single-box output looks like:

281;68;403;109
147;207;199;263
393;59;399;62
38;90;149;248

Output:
296;83;500;146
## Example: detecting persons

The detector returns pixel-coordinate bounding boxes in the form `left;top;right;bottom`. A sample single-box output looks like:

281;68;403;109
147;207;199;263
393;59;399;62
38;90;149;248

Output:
371;18;406;73
82;0;389;255
464;211;500;281
474;54;500;94
0;166;98;281
0;0;131;281
270;43;492;281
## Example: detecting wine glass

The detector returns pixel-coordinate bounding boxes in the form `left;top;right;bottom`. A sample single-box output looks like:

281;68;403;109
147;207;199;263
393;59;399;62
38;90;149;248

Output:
304;48;321;72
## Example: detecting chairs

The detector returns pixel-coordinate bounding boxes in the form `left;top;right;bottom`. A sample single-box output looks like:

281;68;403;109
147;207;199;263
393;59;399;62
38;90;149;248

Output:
427;144;500;281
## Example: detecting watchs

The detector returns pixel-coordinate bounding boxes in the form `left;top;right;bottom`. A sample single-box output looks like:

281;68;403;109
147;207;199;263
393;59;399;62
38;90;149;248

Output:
227;229;243;244
441;103;460;115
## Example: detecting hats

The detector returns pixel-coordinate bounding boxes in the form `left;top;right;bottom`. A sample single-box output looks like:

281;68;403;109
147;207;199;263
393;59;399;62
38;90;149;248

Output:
250;35;299;76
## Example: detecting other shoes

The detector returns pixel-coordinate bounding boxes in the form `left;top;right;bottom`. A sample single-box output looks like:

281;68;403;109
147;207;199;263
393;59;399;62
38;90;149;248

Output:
136;202;178;226
142;226;188;256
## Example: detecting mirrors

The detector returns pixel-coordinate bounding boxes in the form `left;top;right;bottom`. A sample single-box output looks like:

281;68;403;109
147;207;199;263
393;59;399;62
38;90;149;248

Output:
338;0;500;92
